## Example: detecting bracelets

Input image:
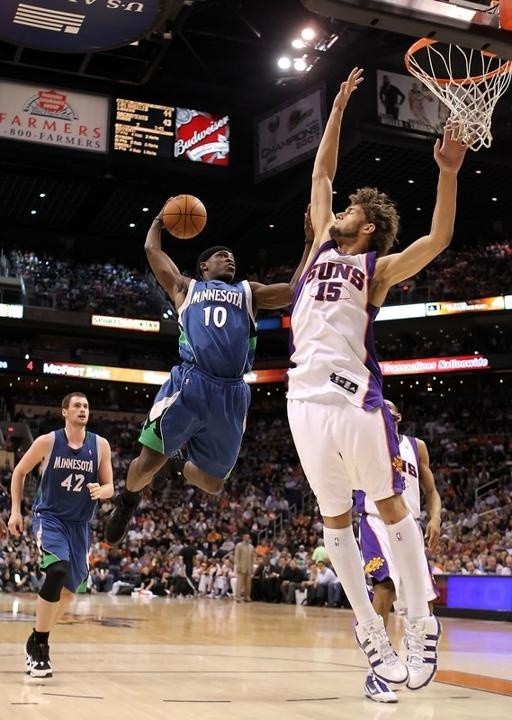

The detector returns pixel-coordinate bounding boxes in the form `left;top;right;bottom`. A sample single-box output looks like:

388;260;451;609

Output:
304;239;313;244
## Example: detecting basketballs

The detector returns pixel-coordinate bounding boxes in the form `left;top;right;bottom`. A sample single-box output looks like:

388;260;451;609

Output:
163;194;208;239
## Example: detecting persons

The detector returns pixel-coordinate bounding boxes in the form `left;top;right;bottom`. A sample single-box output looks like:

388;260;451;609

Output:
413;240;511;301
84;412;340;607
285;68;468;688
380;74;405;120
1;251;177;322
9;393;114;678
395;383;512;575
104;198;314;547
409;83;433;125
246;264;291;318
0;400;66;594
438;88;453;125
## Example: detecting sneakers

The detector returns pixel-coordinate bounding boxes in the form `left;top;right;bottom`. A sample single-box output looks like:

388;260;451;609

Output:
106;489;143;546
355;613;442;703
25;633;53;678
